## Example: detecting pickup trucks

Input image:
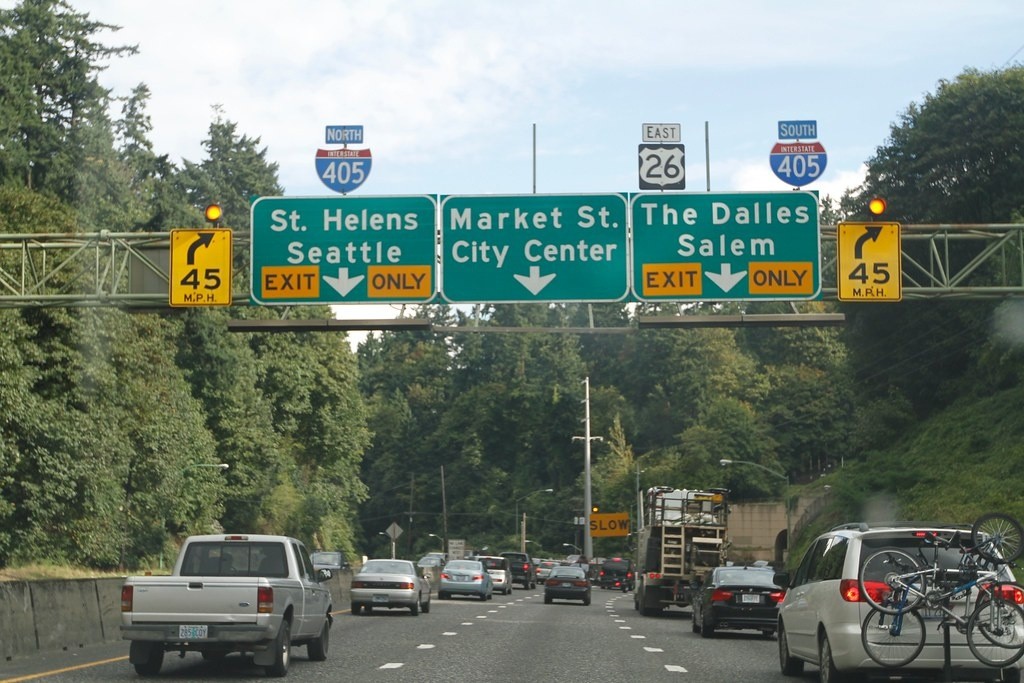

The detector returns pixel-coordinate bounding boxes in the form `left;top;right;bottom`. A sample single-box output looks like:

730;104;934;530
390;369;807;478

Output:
120;535;333;676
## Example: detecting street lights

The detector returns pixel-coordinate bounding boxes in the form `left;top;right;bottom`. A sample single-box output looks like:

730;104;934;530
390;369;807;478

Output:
720;459;789;559
379;532;396;558
515;489;553;552
429;534;444;553
563;543;582;555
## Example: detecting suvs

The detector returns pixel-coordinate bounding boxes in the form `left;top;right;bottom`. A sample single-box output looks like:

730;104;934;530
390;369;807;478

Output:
600;558;634;593
498;552;537;590
779;521;1024;683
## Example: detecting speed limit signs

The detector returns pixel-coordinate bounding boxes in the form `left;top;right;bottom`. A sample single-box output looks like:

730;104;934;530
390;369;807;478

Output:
640;146;686;189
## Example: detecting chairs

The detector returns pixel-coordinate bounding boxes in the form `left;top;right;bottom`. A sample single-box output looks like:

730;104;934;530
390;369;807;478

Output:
199;556;228;575
257;557;285;576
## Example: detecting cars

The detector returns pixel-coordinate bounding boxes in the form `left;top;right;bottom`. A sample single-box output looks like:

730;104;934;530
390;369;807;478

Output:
438;559;493;601
470;556;513;595
311;552;347;572
350;559;432;615
416;553;446;571
544;566;591;605
692;566;786;638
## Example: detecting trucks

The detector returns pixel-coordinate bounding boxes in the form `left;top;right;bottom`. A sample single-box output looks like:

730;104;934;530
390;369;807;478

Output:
634;485;732;617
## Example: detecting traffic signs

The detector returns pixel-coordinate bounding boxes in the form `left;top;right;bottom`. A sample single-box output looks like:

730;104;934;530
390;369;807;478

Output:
170;228;232;307
629;191;822;303
440;193;631;304
835;220;902;304
250;195;437;304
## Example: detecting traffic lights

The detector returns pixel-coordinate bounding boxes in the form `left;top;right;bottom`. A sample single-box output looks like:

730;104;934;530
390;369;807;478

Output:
867;197;886;216
206;206;222;224
591;505;600;513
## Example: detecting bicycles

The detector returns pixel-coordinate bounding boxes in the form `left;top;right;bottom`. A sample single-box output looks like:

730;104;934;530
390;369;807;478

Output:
861;511;1024;667
